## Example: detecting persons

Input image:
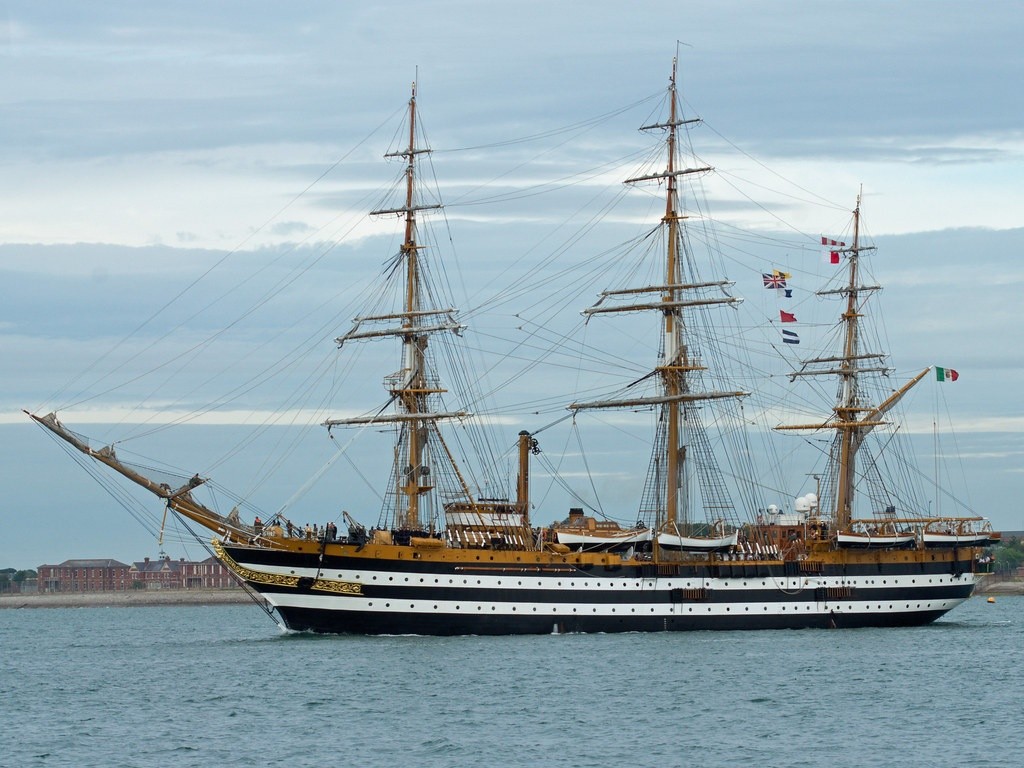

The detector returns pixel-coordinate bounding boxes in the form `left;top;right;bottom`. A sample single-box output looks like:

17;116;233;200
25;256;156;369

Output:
635;552;649;561
298;522;337;540
254;516;280;536
286;520;293;538
788;530;796;542
808;521;830;541
348;524;443;546
979;553;996;573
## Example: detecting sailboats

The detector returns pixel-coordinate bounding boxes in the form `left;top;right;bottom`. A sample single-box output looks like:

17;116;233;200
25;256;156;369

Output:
18;41;1005;637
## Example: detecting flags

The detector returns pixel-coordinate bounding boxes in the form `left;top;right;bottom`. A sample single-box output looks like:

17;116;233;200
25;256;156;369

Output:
780;310;800;324
762;268;794;298
832;252;840;263
935;365;959;382
782;329;801;344
821;237;848;246
